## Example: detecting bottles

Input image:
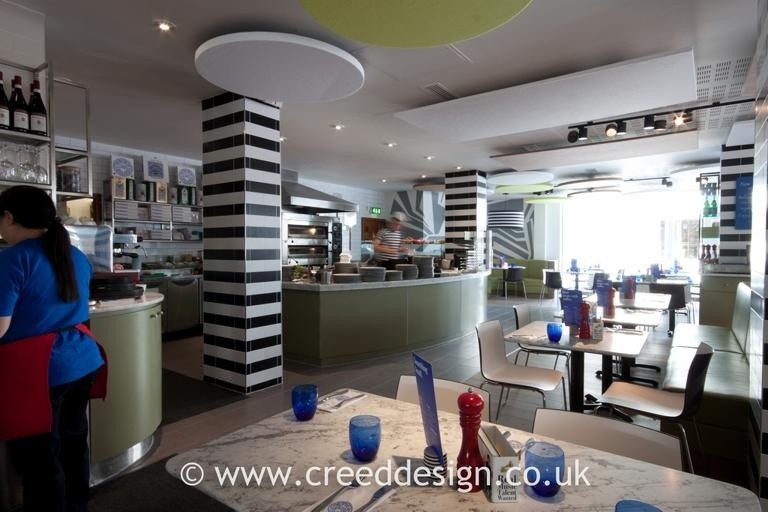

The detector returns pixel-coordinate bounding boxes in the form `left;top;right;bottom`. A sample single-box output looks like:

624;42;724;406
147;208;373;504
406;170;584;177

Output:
625;276;634;299
578;300;591;340
457;386;486;493
605;287;616;317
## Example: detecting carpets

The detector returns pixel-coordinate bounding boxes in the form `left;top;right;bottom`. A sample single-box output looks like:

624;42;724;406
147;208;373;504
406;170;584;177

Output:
11;452;236;512
160;368;249;427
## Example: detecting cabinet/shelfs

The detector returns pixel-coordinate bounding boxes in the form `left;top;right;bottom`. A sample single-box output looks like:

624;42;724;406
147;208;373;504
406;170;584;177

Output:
103;179;203;273
167;278;203;331
700;275;751;328
0;59;56;246
140;279;167;332
46;75;93;197
700;172;720;265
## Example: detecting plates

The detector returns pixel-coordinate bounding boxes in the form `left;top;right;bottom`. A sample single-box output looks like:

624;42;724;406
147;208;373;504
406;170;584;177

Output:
360;266;386;282
397;264;418;278
385;269;403;282
332;262;360;283
411;256;434;279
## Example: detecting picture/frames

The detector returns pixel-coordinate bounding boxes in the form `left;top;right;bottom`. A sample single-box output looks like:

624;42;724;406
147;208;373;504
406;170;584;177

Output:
175;165;196;186
142;155;169;183
110;154;134;180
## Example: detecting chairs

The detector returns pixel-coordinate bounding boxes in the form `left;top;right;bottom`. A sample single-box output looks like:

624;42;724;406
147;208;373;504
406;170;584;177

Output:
636;285;656;333
618;275;636;292
513;305;571;393
532;408;684;472
671;282;750;353
649;284;686;310
475;319;567;420
593;342;713;474
538;269;556;303
506;268;528;300
490;269;503;295
395;375;491;422
540;272;564;309
589;273;605;295
662;324;750;396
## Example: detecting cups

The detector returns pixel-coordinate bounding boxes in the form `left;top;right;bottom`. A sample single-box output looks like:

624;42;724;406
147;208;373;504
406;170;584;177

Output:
291;384;319;421
547;323;562;342
524;441;564;496
349;416;381;462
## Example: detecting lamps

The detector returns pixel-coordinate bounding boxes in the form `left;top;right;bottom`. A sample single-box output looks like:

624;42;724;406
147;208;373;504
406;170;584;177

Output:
153;18;176;33
488;193;524;228
655;120;666;128
456;166;461;170
605;124;617;137
579;128;587;140
668;182;673;186
682;109;693;123
644;116;654;129
663;180;666;185
673;116;682;127
382;179;387;184
617;122;626;133
545;191;549;194
342;212;357;250
330;123;345;130
568;131;578;143
383;142;398;146
421;174;427;178
427;157;431;160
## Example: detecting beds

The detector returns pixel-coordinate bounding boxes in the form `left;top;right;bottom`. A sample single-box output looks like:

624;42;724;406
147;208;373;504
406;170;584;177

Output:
166;387;762;512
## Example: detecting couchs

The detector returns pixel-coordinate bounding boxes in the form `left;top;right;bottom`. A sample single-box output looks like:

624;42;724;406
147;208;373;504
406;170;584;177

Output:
488;259;555;299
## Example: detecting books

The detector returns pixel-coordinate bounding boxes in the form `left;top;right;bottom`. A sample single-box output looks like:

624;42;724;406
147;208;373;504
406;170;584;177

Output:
650;264;659;278
595;278;613;306
412;349;444;467
561;288;583;328
622;276;637;294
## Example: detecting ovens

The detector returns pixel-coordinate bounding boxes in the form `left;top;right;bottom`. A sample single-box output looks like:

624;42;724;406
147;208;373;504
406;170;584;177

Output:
281;212;342;266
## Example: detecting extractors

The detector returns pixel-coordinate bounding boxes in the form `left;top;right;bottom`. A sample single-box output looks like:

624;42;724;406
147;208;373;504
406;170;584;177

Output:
280;180;359;213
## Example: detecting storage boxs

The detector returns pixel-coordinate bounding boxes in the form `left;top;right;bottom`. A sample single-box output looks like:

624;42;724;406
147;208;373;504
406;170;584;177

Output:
589;320;604;340
482;451;521;502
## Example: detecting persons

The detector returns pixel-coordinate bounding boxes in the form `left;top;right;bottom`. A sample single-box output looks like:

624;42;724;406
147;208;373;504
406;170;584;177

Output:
371;211;414;270
0;185;109;511
